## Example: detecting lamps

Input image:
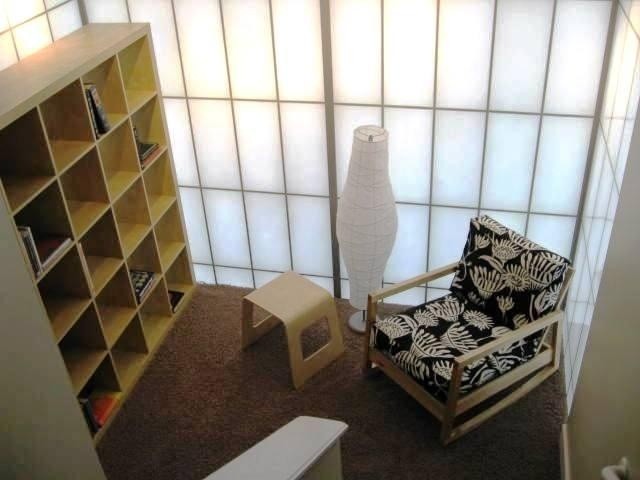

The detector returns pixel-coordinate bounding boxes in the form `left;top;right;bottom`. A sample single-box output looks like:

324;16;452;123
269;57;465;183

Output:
334;124;403;336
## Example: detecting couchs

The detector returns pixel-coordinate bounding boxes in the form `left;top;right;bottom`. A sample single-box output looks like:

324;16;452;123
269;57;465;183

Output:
359;212;577;448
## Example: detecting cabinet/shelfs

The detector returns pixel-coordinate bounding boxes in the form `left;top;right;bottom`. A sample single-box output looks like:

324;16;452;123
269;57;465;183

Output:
1;20;196;446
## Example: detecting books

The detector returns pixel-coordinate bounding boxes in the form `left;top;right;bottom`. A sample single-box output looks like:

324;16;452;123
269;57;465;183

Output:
140;146;163;169
32;229;73;270
83;88;99;140
78;392;120;440
132;126;141;153
84;83;110;134
167;289;185;313
17;224;43;279
138;143;160;162
130;269;156;304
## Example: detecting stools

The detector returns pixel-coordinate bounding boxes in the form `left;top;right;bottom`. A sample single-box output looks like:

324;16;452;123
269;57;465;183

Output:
239;269;344;391
